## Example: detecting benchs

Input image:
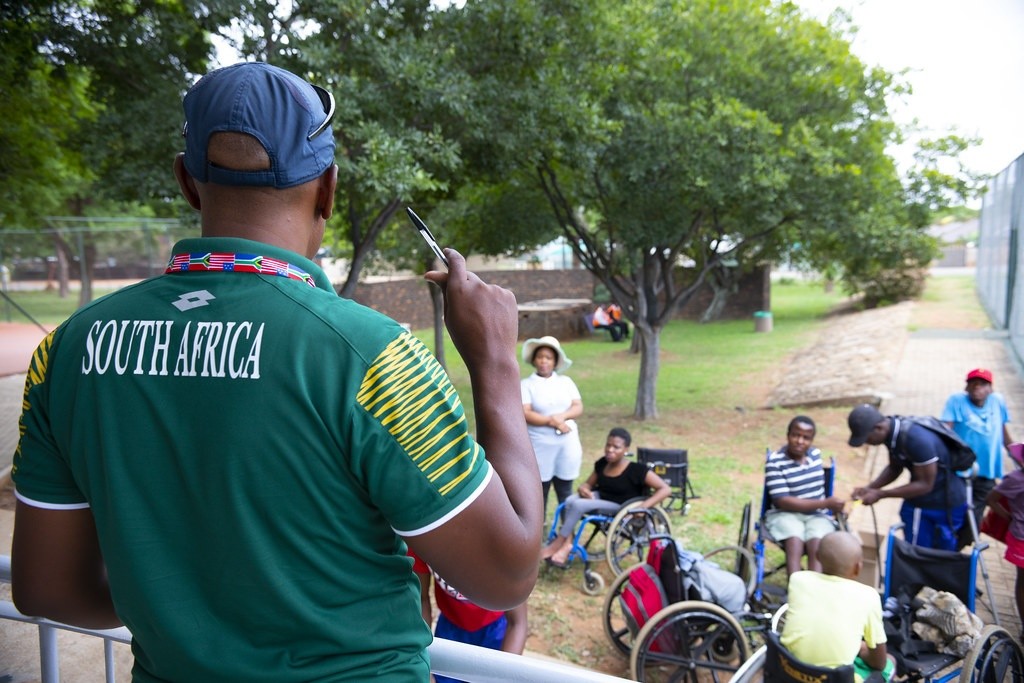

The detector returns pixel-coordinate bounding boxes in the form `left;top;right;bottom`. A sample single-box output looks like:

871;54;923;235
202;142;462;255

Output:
584;314;621;341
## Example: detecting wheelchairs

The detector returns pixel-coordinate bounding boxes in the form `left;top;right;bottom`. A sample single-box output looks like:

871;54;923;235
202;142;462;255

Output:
601;532;775;683
737;447;1024;683
549;452;672;596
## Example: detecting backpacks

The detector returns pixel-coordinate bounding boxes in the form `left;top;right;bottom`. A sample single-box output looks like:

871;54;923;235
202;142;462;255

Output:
619;541;678;655
898;416;975;472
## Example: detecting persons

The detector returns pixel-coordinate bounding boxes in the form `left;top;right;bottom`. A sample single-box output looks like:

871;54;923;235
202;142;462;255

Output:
592;300;630;342
940;369;1014;534
849;403;968;551
986;443;1024;646
541;426;672;563
764;416;849;581
520;336;583;543
780;531;897;683
10;61;544;683
406;547;527;683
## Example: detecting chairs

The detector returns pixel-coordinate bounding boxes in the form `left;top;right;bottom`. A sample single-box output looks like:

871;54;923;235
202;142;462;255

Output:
636;446;701;516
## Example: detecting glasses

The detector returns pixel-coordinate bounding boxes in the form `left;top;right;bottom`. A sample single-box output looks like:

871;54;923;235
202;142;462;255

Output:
182;85;335;141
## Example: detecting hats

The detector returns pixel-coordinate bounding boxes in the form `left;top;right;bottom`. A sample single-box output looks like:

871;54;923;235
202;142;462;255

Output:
185;64;336;185
518;335;572;376
965;369;993;382
847;403;882;448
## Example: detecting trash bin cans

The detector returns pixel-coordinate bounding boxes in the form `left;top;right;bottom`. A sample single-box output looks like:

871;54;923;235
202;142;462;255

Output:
754;311;773;332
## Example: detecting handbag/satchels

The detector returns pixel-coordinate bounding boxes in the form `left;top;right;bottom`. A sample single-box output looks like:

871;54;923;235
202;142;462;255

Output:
979;501;1009;543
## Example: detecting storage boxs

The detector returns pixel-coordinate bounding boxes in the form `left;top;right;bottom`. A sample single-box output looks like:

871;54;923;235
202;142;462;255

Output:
853;532;887;591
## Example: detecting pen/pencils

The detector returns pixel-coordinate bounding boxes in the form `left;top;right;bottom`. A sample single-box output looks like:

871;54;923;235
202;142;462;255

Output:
406;206;450;269
